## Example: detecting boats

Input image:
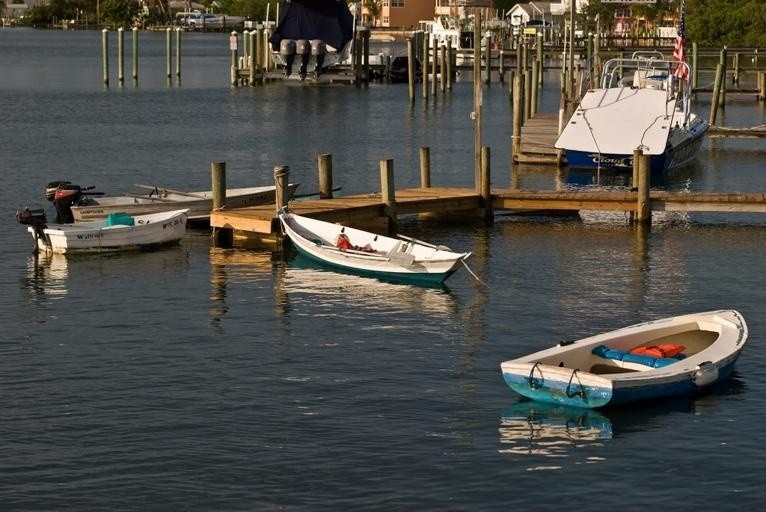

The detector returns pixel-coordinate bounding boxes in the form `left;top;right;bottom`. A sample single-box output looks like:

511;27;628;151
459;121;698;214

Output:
498;306;754;408
22;249;180;300
552;48;712;179
490;379;749;451
13;205;190;252
278;251;462;325
275;205;473;287
37;170;299;232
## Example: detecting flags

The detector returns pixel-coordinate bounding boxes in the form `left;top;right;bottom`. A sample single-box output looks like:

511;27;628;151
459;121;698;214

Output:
671;1;692;83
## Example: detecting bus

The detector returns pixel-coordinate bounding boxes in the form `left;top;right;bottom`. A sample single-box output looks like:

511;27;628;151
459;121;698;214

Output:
169;9;219;27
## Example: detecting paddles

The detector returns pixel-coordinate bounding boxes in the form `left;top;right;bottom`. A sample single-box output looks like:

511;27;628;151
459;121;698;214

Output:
316;242;414;267
397;234;452;252
